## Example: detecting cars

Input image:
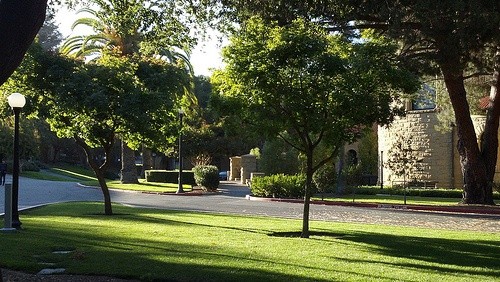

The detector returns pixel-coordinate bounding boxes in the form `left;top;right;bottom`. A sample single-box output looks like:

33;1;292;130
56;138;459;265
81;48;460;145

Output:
218;170;227;181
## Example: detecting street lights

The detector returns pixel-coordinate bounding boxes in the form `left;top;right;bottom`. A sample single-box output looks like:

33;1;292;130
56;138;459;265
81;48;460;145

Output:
7;92;27;226
174;109;186;194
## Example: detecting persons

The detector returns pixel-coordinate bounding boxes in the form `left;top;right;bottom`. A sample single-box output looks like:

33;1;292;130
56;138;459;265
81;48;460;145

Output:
0;159;8;186
